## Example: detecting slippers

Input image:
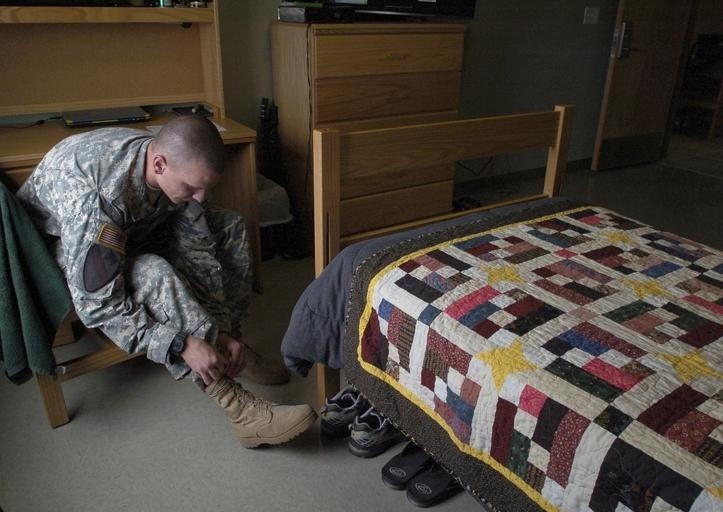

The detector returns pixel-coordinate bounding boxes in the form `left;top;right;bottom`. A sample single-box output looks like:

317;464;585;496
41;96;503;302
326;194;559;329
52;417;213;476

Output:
406;463;465;508
381;442;435;491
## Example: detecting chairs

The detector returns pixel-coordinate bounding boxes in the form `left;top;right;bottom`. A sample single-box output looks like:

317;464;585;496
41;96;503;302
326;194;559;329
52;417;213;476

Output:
0;179;144;430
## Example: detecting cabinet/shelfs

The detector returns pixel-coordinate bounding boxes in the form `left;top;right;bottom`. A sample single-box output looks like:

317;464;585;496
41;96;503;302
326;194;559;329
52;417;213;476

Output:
270;18;471;263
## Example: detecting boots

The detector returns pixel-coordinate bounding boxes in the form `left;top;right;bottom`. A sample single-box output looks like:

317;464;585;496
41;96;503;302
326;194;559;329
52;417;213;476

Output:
204;373;319;450
230;341;292;385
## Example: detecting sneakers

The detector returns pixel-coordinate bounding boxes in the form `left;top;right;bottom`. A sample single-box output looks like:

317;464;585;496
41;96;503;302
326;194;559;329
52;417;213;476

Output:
348;406;409;459
318;385;370;439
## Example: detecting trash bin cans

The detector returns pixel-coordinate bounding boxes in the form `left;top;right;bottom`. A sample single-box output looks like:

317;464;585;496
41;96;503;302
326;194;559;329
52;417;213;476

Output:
256;172;295;262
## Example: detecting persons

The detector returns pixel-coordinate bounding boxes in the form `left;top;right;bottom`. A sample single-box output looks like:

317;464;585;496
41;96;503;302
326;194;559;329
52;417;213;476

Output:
12;114;322;451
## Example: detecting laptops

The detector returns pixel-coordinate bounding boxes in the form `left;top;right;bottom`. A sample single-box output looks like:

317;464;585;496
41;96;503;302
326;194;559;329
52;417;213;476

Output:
61;106;151;127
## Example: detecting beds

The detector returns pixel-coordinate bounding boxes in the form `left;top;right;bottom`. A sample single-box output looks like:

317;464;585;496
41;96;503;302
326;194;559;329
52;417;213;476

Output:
280;101;722;512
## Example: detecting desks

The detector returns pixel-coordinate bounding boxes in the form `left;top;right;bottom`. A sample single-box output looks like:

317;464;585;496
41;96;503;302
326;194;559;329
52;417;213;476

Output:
1;0;265;292
